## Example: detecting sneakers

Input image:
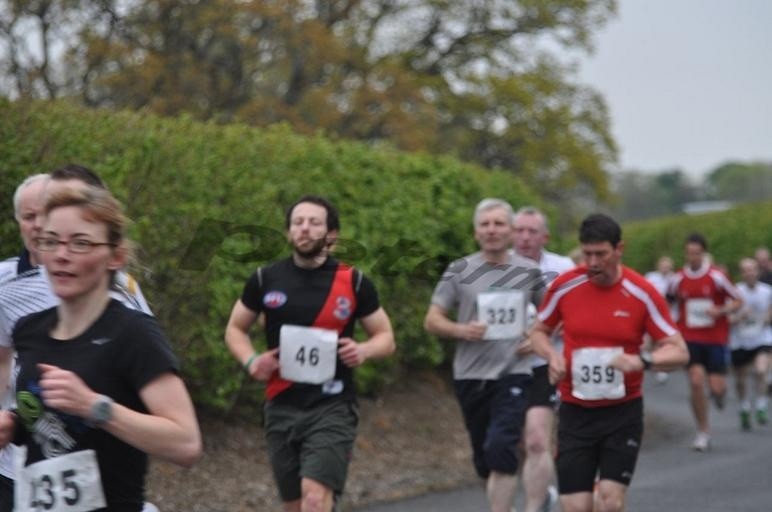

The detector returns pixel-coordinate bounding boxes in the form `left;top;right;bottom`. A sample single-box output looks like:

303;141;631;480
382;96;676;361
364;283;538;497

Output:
739;409;768;430
689;431;712;452
655;368;669;388
714;398;725;411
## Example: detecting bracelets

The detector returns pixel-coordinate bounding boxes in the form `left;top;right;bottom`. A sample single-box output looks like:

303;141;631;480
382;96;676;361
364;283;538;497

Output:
244;349;262;369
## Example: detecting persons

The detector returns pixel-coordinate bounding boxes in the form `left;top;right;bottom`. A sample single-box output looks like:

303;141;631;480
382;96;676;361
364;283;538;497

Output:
0;179;204;511
224;195;398;512
0;165;157;510
0;172;152;317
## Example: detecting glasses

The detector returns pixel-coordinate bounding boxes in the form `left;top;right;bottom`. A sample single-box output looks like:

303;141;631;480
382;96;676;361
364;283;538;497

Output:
31;236;119;255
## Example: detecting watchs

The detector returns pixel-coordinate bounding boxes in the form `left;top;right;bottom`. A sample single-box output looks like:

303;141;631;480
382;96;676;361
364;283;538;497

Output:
86;394;112;429
